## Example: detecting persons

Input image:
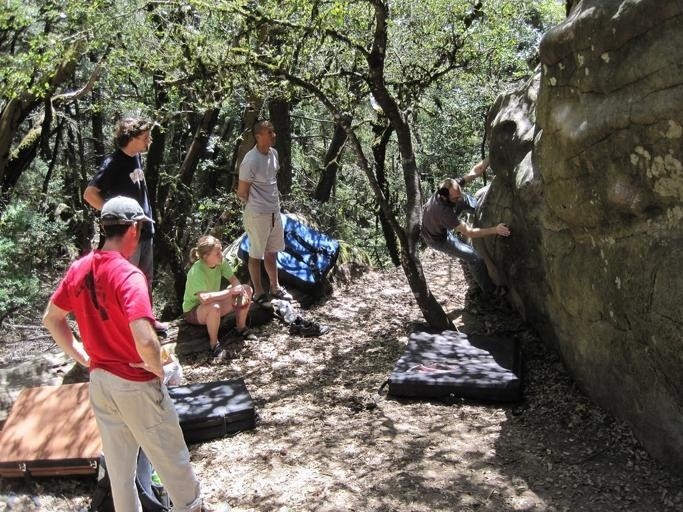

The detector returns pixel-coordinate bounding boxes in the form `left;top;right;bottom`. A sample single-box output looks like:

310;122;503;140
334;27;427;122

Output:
179;233;255;360
235;116;296;308
81;117;169;334
40;193;212;512
420;154;512;299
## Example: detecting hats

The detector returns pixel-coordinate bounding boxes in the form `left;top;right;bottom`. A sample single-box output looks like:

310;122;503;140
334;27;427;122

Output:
101;195;156;225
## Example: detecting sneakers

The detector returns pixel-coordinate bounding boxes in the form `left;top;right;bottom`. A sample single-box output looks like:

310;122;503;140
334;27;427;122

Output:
232;326;257;340
289;317;330;338
201;502;232;512
208;343;226;357
253;293;271;308
154;321;168;332
269;286;293;300
